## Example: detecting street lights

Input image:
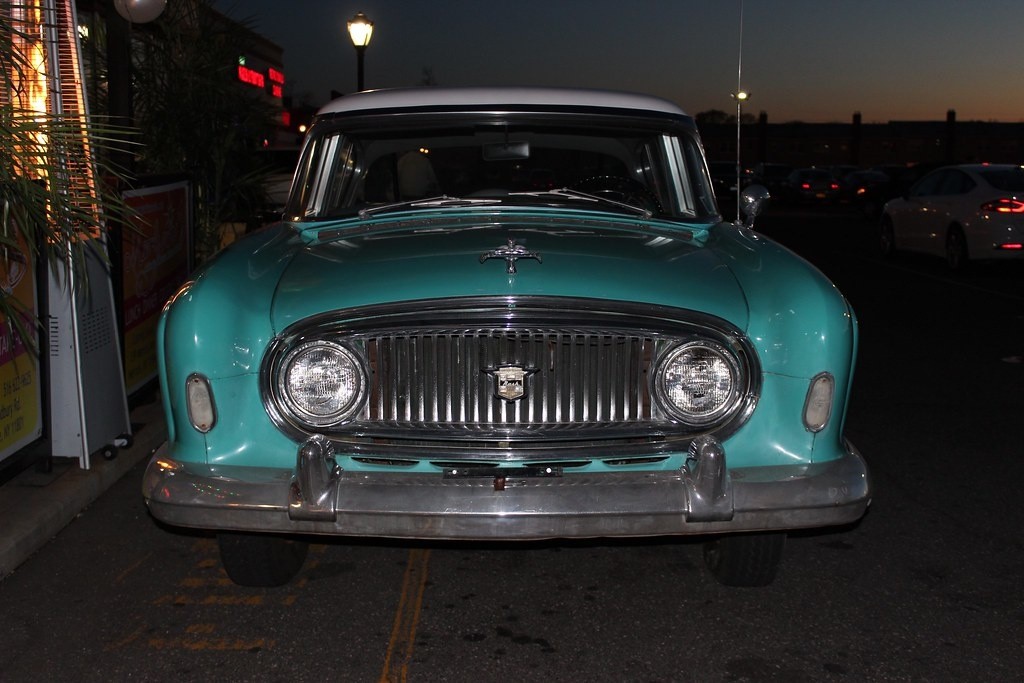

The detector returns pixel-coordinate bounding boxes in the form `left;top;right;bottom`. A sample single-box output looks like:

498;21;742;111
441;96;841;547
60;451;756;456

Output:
346;12;375;91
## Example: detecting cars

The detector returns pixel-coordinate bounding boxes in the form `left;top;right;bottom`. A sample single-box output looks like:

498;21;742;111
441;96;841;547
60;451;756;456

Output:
142;84;873;588
845;171;880;202
712;175;737;192
879;164;1024;269
788;168;841;205
744;169;784;200
